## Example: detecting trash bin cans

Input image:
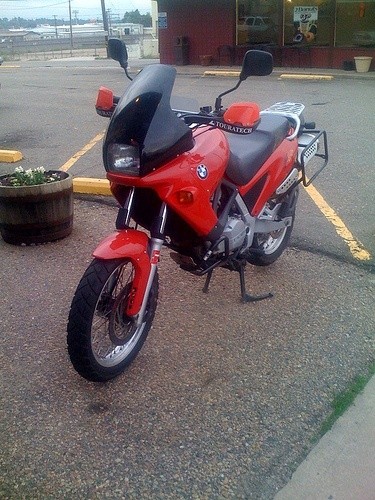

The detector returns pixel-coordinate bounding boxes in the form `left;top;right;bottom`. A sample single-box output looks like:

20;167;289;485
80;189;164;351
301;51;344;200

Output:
174;35;189;67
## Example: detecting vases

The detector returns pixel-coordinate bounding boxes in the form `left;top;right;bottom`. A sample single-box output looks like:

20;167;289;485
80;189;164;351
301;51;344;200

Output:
0;163;73;245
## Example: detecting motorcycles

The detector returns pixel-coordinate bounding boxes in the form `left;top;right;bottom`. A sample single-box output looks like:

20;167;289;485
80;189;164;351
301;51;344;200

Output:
66;38;329;382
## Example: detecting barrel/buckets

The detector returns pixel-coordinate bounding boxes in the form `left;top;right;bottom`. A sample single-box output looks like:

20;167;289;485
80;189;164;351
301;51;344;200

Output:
354;56;373;73
